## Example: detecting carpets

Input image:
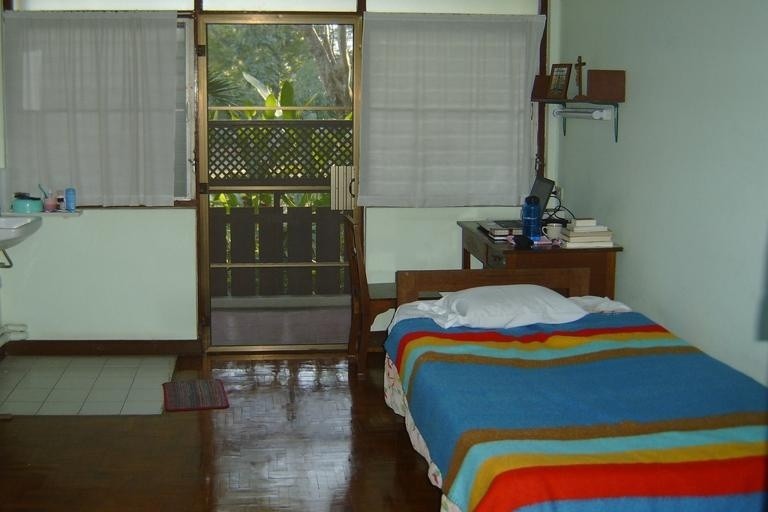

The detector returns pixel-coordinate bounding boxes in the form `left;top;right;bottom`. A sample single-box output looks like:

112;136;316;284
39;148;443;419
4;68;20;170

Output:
162;378;229;411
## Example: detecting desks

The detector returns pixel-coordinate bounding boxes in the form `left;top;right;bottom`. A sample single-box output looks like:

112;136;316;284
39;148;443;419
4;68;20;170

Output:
457;220;622;300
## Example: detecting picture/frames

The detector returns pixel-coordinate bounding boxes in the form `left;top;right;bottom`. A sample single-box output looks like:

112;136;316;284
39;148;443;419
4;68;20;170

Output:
545;64;572;98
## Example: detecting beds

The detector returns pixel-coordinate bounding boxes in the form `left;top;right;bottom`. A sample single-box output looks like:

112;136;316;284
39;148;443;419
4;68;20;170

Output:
383;267;768;512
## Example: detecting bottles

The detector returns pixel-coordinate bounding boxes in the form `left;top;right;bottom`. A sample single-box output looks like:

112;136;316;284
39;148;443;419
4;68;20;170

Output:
57;187;76;212
521;195;542;241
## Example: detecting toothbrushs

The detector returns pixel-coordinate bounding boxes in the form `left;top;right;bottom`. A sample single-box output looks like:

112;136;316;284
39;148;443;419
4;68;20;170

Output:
38;184;48;198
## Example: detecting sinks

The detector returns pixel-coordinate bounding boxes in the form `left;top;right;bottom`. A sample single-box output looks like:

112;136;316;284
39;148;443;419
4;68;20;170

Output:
0;215;42;251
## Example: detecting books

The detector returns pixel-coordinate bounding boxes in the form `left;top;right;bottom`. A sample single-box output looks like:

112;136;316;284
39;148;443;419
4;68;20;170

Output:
557;218;614;249
488;227;525;236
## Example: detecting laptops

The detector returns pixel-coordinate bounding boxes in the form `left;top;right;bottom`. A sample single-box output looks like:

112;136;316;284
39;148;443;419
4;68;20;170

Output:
477;175;555;232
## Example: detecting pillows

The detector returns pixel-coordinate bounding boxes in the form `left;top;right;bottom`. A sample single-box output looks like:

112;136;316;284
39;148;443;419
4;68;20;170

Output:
440;284;590;329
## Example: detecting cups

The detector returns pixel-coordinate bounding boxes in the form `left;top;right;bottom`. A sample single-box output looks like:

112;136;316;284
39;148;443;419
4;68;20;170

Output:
43;197;57;212
542;223;563;240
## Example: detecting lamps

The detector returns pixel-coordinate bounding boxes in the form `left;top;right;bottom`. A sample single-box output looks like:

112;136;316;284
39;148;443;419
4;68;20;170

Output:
553;108;611;120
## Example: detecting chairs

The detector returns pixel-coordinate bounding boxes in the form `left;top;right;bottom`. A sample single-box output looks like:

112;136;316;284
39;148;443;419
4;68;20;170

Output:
343;214;442;365
